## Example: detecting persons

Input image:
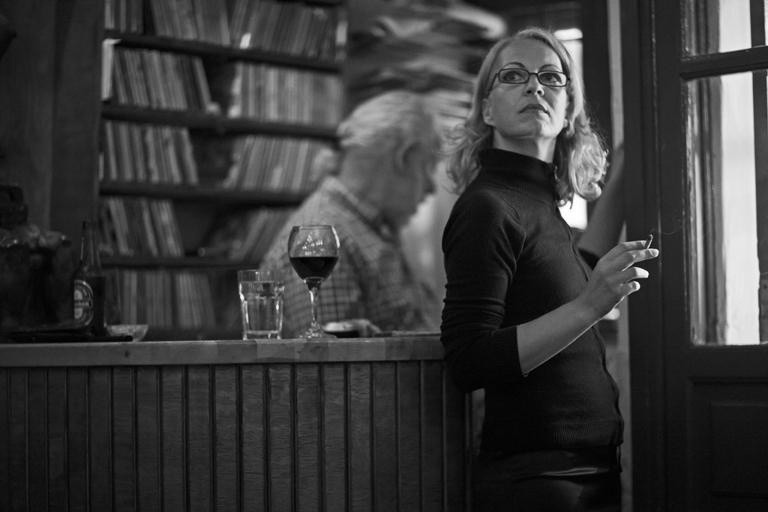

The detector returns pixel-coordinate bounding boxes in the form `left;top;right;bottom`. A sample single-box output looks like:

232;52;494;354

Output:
435;27;662;510
255;86;444;335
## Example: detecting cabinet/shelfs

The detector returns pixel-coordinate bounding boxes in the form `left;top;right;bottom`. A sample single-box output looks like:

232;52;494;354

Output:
44;2;352;338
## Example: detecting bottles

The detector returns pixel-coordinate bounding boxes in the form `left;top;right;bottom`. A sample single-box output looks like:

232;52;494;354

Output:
72;223;107;337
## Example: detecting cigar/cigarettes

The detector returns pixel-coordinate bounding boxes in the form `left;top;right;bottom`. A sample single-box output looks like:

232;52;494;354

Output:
644;234;654;248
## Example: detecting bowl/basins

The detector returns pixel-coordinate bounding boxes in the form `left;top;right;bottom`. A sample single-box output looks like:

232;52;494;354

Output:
105;323;150;341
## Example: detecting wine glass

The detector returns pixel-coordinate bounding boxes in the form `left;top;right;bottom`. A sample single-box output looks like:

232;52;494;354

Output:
287;225;341;340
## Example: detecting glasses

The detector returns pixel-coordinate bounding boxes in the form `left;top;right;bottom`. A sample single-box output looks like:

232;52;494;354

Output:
486;67;571;98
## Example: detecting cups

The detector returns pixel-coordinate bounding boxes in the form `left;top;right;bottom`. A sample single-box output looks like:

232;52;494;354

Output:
238;269;286;342
